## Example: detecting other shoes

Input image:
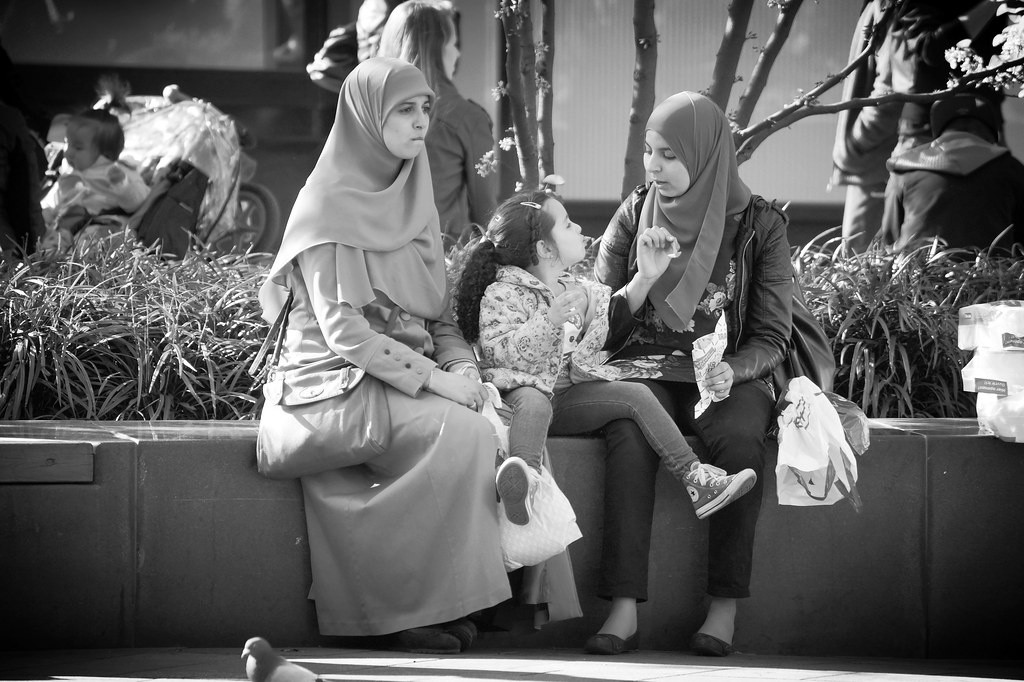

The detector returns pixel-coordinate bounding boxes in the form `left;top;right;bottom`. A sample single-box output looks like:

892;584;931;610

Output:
375;621;477;654
584;630;640;653
689;628;736;657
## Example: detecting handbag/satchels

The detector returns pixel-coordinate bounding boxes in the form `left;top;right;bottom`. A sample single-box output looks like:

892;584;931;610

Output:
774;374;865;512
256;366;391;480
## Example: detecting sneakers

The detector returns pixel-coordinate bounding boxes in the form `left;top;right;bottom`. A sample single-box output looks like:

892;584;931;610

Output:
683;461;757;519
495;457;538;525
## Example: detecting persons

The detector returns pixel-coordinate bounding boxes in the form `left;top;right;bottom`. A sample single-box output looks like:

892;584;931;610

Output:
830;0;1024;259
260;58;583;651
585;90;796;656
374;0;508;259
0;48;55;268
454;188;757;528
306;21;359;138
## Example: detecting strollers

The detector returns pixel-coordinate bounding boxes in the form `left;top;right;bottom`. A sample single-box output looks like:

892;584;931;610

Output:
0;84;280;266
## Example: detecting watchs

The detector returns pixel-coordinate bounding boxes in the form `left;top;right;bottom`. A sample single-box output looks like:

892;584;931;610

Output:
41;78;151;248
